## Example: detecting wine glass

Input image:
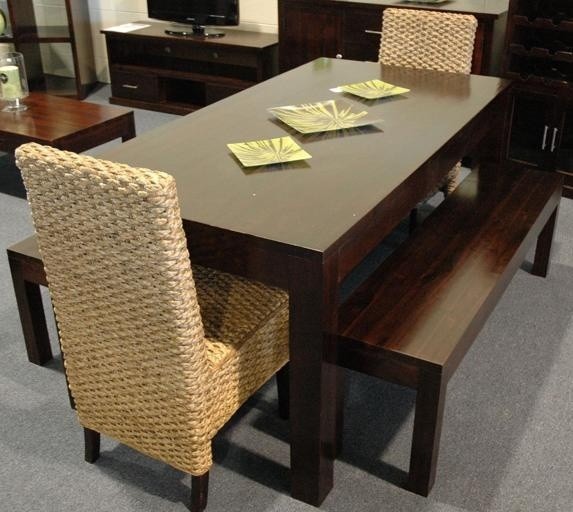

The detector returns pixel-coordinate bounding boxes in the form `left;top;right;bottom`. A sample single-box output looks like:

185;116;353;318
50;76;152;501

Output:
0;51;32;114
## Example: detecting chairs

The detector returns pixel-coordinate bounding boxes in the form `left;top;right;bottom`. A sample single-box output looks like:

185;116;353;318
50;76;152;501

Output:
13;142;293;512
347;8;480;252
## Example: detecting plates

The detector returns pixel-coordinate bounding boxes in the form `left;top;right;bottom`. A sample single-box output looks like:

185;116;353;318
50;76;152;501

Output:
336;78;411;100
266;95;385;134
224;134;313;170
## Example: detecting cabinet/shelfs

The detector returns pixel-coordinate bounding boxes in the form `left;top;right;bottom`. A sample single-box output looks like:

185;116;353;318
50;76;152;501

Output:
276;1;503;169
0;1;97;101
492;1;573;199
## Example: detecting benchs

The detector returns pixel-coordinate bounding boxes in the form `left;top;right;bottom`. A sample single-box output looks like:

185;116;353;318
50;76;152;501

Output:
5;234;50;367
324;157;567;499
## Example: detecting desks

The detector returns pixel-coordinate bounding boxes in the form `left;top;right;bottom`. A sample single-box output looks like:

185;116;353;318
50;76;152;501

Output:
0;91;136;155
76;57;518;507
98;20;278;117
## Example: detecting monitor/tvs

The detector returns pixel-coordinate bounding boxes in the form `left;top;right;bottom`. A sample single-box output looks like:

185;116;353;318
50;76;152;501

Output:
147;0;240;37
